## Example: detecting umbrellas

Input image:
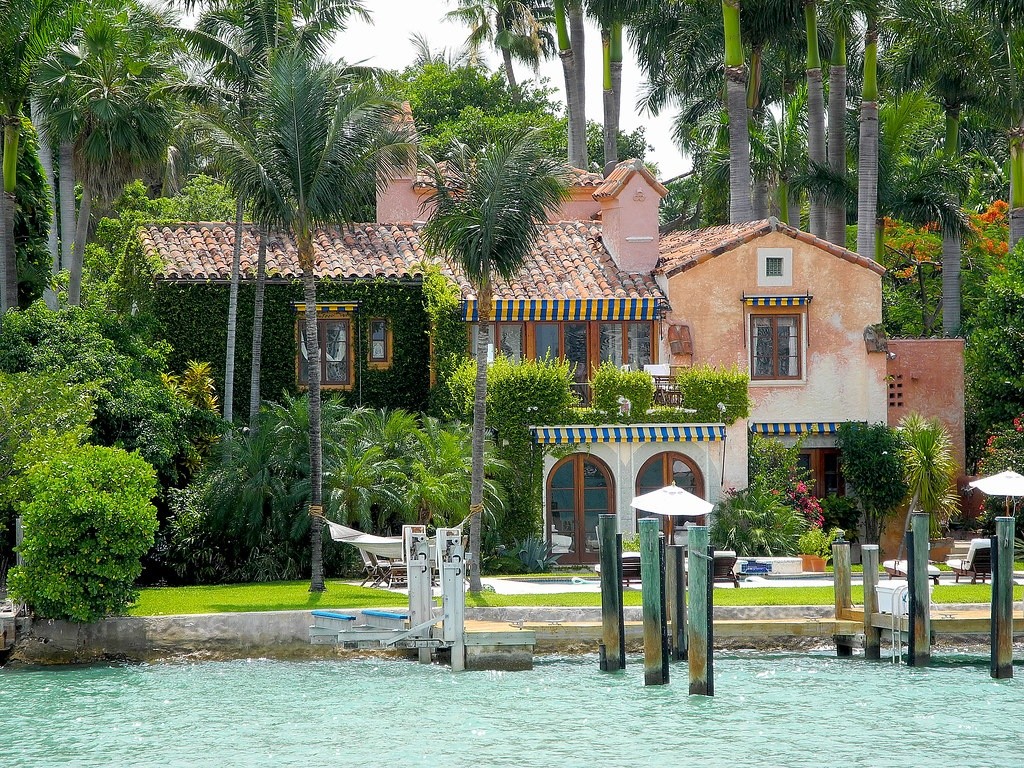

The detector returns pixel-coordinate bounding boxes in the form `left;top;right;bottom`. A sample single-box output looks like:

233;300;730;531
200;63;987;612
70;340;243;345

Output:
630;480;714;544
968;470;1024;518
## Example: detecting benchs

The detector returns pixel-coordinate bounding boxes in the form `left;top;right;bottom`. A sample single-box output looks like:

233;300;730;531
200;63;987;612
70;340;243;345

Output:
311;611;357;633
883;559;942;586
361;609;409;632
595;550;741;588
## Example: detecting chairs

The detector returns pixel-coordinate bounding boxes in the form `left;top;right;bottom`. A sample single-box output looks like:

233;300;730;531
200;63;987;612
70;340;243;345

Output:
355;533;473;590
946;538;992;584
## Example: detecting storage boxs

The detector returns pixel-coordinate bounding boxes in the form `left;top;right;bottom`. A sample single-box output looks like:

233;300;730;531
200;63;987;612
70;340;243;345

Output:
874;579;935;615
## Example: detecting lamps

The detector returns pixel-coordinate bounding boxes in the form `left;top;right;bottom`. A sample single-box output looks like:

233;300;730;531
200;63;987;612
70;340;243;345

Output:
886;349;897;361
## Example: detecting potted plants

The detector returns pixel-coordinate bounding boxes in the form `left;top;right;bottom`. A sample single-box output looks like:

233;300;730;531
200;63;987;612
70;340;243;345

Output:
935;483;963;539
796;525;847;572
